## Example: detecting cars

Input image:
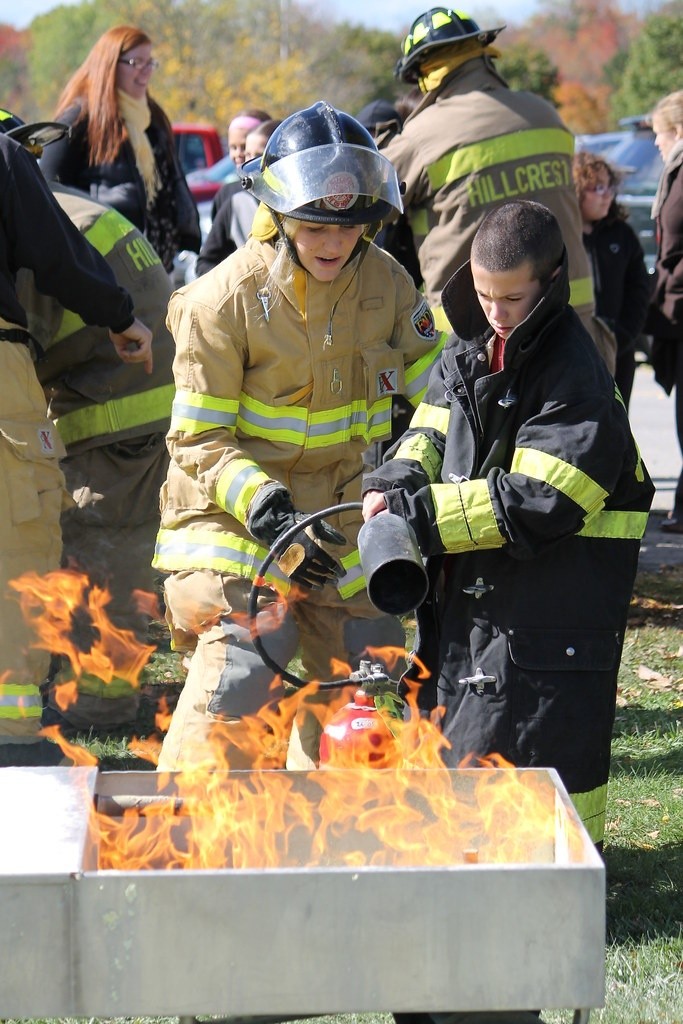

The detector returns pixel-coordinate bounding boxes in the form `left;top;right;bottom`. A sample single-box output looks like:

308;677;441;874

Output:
169;125;225;245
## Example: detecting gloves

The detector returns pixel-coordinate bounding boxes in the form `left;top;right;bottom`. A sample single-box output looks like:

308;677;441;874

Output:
250;489;347;591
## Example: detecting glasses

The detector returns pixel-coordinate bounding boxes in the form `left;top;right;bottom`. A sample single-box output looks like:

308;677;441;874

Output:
585;184;615;196
118;57;159;72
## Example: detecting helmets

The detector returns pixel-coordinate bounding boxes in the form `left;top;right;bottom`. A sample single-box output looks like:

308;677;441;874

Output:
394;7;507;84
236;101;406;224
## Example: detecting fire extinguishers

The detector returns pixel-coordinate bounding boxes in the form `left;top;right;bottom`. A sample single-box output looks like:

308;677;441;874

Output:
249;497;430;768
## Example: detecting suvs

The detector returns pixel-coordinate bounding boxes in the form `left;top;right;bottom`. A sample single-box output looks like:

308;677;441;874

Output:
602;115;665;283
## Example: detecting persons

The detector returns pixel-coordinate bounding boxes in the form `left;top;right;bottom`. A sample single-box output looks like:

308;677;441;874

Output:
0;130;152;766
363;200;656;853
572;152;648;415
196;87;425;294
40;24;202;271
375;7;618;379
152;101;447;772
648;89;683;532
0;109;176;736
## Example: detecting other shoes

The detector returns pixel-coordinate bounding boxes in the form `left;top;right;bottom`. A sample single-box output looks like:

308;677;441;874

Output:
661;518;683;531
40;707;149;737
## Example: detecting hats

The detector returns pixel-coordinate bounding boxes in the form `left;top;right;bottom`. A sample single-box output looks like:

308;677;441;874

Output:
357;102;397;129
